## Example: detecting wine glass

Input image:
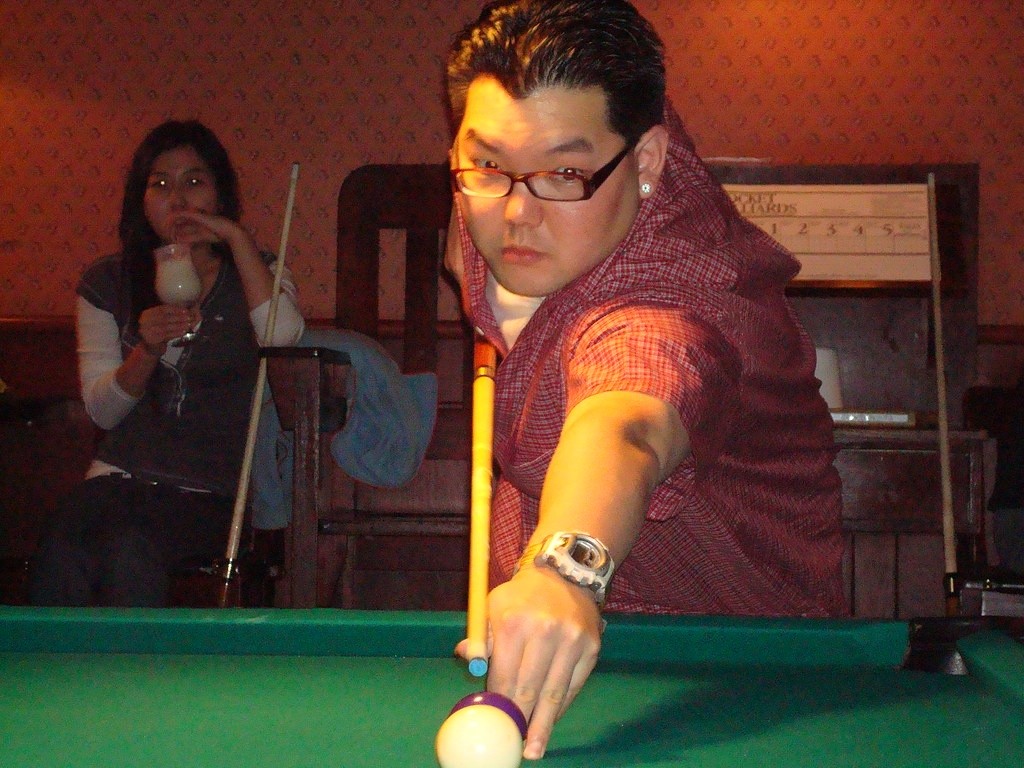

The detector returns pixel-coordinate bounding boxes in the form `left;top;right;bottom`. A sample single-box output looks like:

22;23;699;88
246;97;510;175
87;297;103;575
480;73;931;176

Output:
153;242;203;347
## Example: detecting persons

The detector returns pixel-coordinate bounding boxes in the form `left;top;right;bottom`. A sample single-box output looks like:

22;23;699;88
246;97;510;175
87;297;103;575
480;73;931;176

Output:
444;0;850;759
24;119;302;609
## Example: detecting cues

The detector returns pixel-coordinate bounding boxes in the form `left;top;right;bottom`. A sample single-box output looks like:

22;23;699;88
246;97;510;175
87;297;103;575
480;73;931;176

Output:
924;167;963;618
464;325;500;680
215;157;302;608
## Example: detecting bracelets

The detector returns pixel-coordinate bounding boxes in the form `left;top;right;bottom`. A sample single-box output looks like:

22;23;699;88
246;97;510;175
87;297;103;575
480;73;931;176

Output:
141;340;166;357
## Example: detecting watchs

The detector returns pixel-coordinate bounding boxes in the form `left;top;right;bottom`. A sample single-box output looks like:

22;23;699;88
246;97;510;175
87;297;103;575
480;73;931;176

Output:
513;531;615;602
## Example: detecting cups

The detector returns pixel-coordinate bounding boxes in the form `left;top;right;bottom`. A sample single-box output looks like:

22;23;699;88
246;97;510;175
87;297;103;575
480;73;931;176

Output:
816;346;844;412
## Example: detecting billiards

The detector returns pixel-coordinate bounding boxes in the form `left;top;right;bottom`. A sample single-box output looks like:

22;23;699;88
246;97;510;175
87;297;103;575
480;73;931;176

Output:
433;688;529;768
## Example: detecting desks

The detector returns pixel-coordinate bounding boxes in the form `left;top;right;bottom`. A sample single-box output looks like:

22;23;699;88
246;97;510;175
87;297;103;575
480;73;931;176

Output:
0;606;1024;768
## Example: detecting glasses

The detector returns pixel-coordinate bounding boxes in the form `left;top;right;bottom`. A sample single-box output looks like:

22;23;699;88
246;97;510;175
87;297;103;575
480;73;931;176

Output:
448;134;640;203
147;171;215;191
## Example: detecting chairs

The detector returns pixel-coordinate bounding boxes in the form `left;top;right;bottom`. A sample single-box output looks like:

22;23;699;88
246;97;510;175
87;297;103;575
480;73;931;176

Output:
255;162;474;605
